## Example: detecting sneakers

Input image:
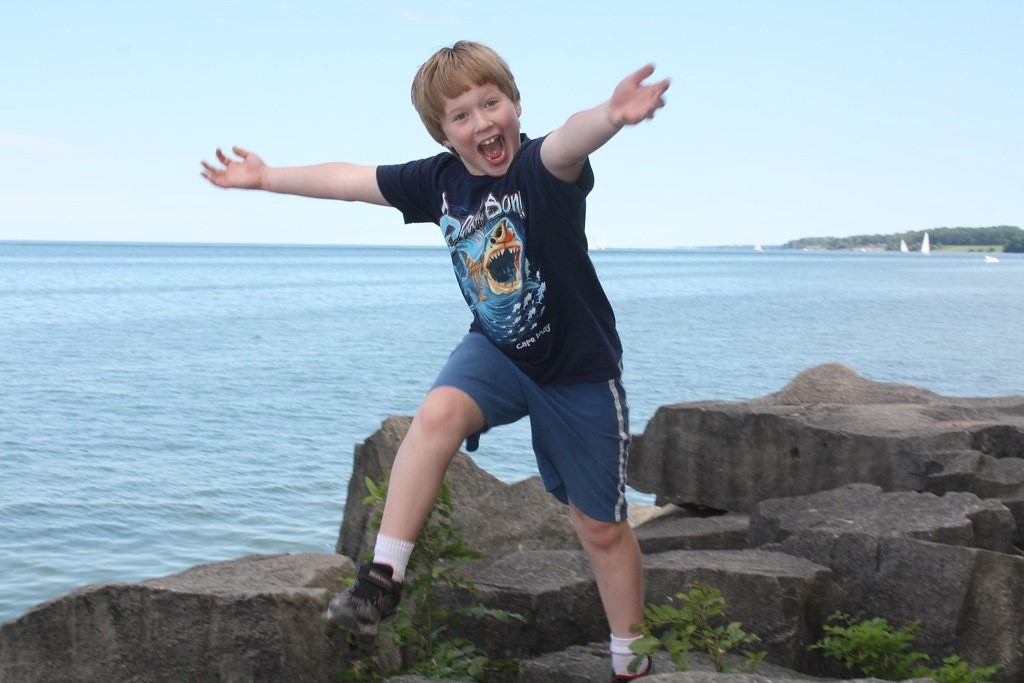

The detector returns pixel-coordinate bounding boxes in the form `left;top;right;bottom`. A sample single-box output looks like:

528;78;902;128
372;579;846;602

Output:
326;561;403;636
611;654;655;683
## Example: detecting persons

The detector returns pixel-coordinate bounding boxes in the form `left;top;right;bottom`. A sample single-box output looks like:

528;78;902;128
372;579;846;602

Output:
201;40;670;683
